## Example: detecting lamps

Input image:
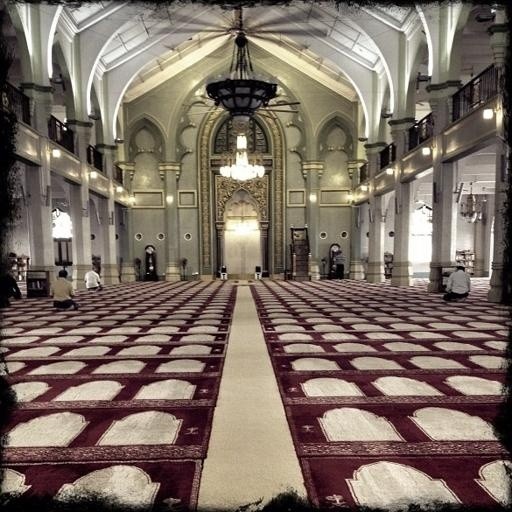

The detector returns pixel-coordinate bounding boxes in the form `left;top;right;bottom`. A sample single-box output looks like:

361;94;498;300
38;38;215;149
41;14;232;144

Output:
219;132;266;182
206;9;278;115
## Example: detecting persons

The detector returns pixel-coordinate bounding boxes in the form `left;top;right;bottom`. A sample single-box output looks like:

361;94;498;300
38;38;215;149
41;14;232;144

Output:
334;250;346;279
49;270;80;312
443;266;471;302
84;266;101;292
0;249;23;307
